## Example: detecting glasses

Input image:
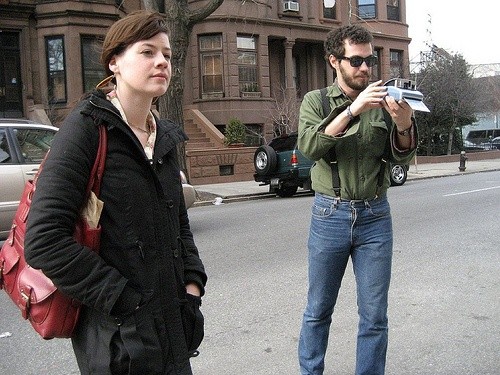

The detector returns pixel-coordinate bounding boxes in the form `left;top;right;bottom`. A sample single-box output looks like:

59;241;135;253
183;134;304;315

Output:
341;54;379;67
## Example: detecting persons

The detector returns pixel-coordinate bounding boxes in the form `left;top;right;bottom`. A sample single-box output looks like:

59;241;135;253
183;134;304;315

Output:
23;10;207;375
299;25;419;375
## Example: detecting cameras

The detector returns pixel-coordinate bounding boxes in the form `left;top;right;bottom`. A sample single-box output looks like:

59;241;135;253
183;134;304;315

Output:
383;77;431;112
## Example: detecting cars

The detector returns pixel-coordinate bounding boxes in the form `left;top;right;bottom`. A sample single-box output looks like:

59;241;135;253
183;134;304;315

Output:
1;118;197;240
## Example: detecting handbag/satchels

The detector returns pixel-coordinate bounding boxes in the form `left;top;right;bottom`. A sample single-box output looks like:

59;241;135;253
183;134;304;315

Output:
0;118;108;341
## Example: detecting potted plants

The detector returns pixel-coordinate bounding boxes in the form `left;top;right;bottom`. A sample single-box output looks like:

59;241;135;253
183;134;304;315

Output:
223;117;245;147
241;82;262;98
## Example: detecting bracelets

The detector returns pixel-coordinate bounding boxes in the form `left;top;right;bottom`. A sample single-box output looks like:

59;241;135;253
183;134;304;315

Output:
347;106;354;121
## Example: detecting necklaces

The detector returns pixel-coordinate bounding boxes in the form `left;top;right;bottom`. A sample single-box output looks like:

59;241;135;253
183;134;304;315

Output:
129;122;151;136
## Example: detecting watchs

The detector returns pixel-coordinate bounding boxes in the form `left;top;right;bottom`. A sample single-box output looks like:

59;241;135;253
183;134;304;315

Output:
397;124;412;136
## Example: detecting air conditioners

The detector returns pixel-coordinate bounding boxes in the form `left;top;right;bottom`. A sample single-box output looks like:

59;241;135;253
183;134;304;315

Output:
283;1;300;11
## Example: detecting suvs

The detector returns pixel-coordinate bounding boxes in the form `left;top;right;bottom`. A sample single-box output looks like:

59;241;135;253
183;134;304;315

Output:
253;130;411;197
467;128;500;150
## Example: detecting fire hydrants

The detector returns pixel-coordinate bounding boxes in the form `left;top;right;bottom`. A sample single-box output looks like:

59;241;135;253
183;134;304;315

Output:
458;151;468;171
